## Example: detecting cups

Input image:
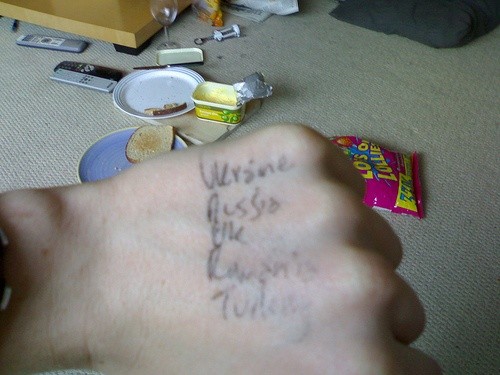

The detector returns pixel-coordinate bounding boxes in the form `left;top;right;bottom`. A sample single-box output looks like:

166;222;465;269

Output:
191;81;246;127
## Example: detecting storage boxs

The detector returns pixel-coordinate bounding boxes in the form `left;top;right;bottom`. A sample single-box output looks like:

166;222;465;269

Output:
191;81;247;126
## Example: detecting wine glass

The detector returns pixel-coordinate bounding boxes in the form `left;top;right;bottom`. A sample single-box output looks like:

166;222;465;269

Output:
148;0;181;51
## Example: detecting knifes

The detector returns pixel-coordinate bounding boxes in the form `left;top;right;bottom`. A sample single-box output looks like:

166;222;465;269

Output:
133;60;204;70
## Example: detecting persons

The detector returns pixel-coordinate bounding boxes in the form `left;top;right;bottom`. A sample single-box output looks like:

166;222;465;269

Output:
0;125;438;375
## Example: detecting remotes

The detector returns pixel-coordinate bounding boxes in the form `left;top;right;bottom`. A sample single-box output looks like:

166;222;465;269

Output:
56;60;122;82
18;35;87;53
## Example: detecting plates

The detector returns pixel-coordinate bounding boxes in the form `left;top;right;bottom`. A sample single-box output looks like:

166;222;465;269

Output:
112;66;205;121
76;127;189;184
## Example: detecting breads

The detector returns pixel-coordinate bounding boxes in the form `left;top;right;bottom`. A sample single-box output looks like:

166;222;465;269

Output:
125;124;174;163
143;101;187;116
